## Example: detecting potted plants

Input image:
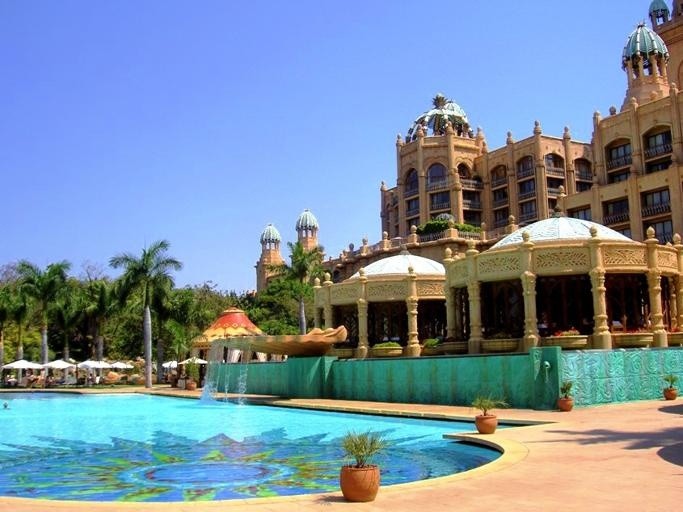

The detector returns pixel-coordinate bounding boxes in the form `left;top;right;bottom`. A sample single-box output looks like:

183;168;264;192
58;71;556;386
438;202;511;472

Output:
479;326;520;353
556;380;574;411
663;372;679;400
184;351;200;390
340;423;384;503
420;337;441;355
470;389;511;434
372;341;404;358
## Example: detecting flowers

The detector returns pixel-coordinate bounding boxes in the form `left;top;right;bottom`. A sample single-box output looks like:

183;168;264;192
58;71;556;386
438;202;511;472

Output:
549;326;580;336
623;329;641;333
668;326;680;332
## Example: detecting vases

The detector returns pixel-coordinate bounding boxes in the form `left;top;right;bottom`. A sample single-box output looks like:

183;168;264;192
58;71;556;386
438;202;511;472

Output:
545;335;589;348
667;331;683;347
611;333;654;348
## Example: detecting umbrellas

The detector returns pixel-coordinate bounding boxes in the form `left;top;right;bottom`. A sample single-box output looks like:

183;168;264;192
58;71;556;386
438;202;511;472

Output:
0;355;207;386
